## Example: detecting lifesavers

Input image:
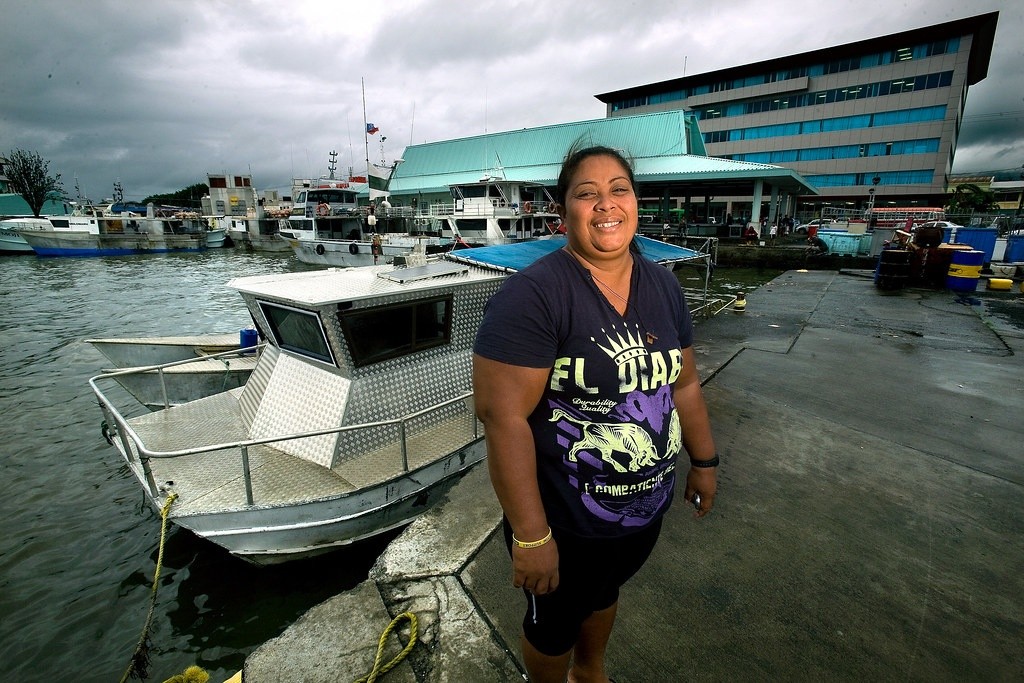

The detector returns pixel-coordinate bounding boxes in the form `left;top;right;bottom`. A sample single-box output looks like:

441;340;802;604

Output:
315;204;329;216
549;202;556;213
524;201;532;214
316;244;325;255
349;243;358;255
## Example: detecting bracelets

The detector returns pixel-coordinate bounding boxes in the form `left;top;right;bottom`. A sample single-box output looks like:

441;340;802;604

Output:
513;526;552;549
690;454;719;468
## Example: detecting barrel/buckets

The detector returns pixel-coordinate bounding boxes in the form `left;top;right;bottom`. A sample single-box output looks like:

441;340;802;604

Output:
240;328;258;353
875;227;987;293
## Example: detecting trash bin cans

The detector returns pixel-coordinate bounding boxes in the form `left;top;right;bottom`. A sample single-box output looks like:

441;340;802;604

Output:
945;250;987;291
816;229;873;258
1002;235;1024;262
876;250;913;296
941;227;953;244
952;228;999;264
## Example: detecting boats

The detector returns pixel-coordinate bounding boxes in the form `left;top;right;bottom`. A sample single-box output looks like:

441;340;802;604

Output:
81;331;265;415
88;235;714;569
0;185;563;268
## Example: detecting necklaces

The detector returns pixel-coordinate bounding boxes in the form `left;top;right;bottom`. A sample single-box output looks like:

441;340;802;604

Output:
570;253;659;344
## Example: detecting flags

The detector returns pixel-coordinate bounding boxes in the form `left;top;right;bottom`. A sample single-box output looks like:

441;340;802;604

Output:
367;161;391;200
349;176;366;188
367;123;379;136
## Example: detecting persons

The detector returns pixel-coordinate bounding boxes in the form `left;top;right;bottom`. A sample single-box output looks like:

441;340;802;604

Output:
679;214;686;234
40;225;46;230
829;217;837;225
86;208;93;215
472;129;718;683
453;234;461;242
280;206;289;210
726;211;796;245
129;212;137;227
803;208;849;221
370;202;375;215
99;199;108;204
311;194;324;200
811;235;829;253
652;210;673;228
